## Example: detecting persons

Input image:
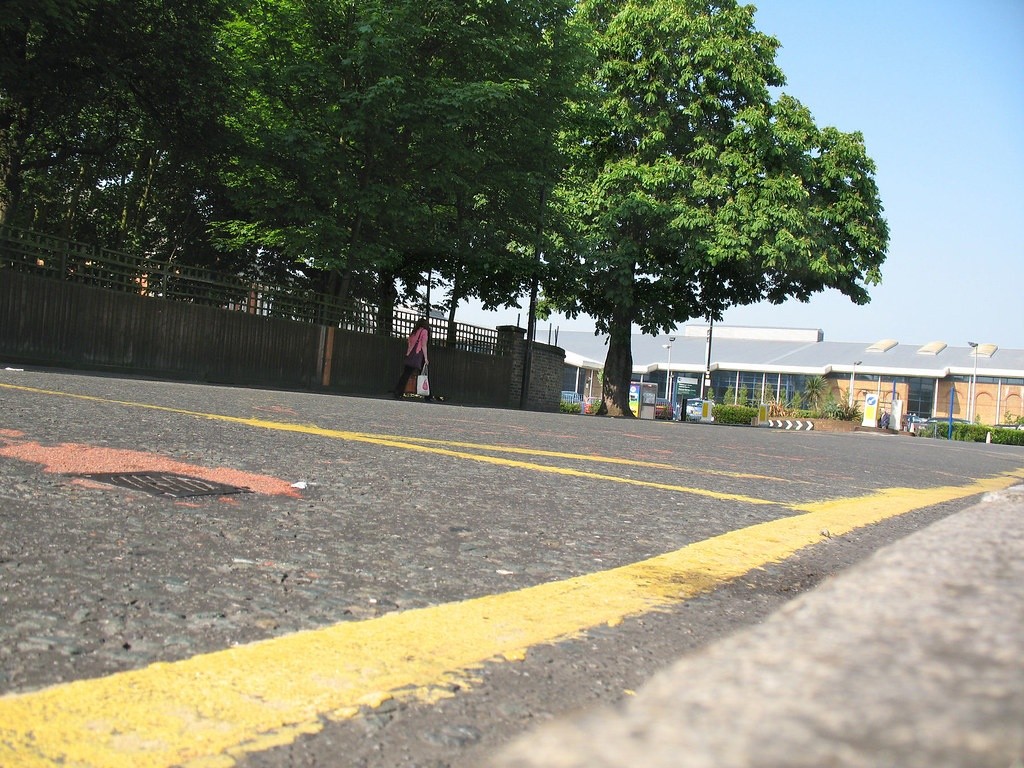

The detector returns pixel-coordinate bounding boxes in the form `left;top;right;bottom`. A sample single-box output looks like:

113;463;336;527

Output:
392;319;436;403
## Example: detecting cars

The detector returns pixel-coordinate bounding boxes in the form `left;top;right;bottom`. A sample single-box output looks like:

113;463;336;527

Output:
656;398;674;420
994;424;1024;431
919;417;965;436
686;397;705;421
878;413;922;432
561;390;579;403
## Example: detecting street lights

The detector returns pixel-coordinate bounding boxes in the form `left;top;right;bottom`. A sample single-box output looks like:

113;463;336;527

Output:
850;360;862;414
662;336;677;400
967;341;979;426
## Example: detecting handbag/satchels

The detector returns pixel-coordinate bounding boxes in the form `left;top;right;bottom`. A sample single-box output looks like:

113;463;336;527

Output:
405;375;417;395
403;348;424;370
416;363;430;396
